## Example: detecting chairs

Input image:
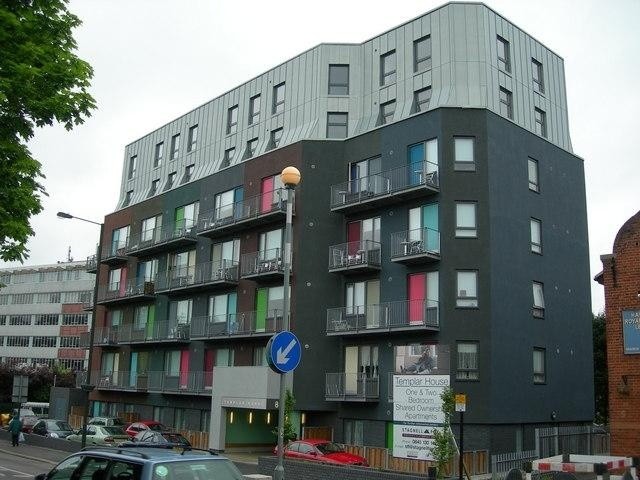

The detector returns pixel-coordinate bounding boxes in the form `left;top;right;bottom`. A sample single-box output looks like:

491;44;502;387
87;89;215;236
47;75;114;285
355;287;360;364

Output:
331;170;439;268
330;318;352;334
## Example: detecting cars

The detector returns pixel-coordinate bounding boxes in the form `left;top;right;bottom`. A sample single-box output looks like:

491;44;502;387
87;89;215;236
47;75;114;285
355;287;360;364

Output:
1;401;193;453
274;438;370;468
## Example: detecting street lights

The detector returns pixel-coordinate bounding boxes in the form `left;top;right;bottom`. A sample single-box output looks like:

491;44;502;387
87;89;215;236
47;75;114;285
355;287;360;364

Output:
273;165;301;478
57;212;105;450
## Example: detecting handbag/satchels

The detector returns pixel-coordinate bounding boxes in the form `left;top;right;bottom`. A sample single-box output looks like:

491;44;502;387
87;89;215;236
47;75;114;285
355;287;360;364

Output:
18;431;25;442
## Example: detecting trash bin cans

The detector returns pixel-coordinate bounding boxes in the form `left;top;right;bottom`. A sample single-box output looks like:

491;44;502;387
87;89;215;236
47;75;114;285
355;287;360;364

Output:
428;466;436;480
526;461;532;473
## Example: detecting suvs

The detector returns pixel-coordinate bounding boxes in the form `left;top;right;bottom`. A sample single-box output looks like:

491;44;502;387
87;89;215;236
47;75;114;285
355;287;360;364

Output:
34;443;245;479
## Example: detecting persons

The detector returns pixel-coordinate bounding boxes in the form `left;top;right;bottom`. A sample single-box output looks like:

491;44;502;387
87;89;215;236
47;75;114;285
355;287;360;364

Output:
8;415;21;446
